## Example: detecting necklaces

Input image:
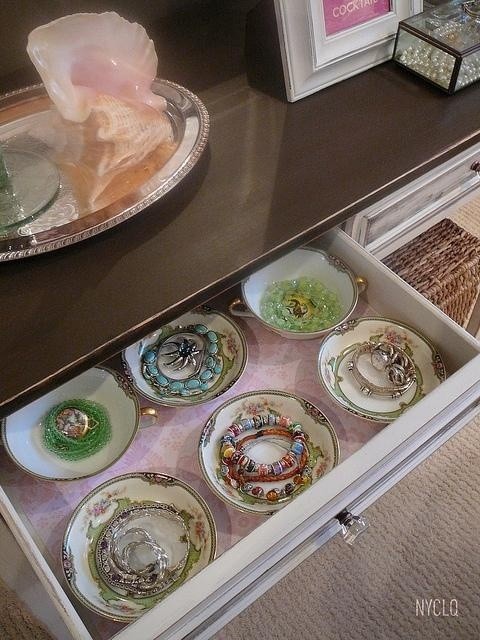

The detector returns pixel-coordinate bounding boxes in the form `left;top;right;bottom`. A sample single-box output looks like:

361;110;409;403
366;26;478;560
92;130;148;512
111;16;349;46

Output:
398;15;480;91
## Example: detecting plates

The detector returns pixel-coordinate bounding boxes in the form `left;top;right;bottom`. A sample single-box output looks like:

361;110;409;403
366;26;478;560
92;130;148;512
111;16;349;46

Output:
317;316;448;425
61;472;231;624
1;364;159;481
230;244;368;341
121;304;250;408
197;389;342;515
0;75;211;264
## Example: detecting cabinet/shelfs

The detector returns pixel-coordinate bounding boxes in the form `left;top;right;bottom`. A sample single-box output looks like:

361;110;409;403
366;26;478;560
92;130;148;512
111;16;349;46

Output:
0;1;480;640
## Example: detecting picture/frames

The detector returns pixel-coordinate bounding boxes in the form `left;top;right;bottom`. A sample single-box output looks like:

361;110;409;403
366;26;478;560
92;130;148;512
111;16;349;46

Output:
274;1;425;103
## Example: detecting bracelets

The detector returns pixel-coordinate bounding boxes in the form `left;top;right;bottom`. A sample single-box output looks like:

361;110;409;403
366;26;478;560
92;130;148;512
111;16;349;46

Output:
141;323;220;392
218;413;309;473
219;426;313;506
348;339;422;400
94;502;192;600
41;398;111;463
259;275;344;335
